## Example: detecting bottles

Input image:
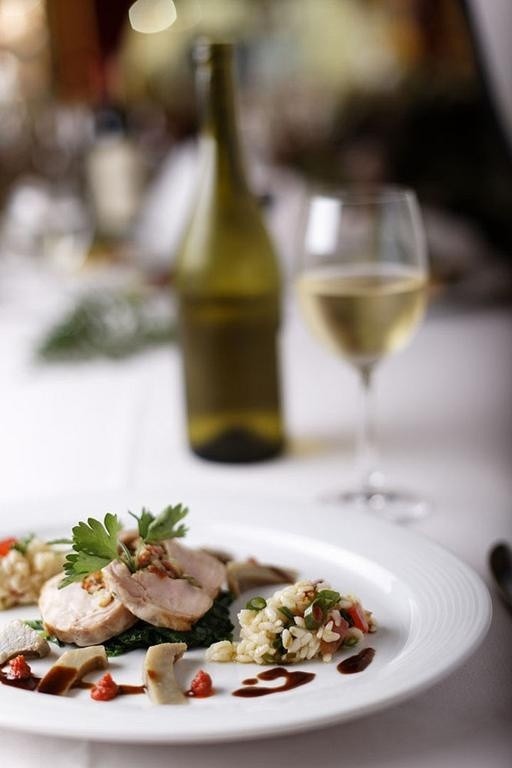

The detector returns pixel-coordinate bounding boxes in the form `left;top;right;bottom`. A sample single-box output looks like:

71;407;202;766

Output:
164;38;287;467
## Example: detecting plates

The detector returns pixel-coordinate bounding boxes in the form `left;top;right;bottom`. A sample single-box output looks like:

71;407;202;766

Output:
1;506;492;745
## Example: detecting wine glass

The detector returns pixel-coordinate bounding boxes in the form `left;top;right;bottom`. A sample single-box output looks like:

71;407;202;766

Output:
299;186;439;506
